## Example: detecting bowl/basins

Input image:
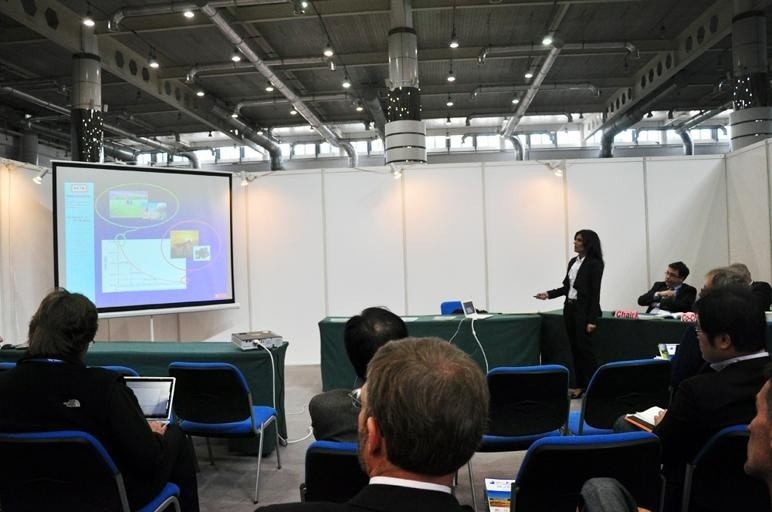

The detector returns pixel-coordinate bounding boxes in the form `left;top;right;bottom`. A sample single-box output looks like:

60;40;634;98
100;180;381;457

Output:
657;343;679;359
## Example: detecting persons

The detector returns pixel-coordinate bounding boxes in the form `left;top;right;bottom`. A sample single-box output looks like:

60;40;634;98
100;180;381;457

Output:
574;363;772;512
729;264;754;288
308;308;409;441
638;261;697;313
0;289;199;512
751;281;772;312
254;337;490;511
654;269;751;376
613;289;771;443
534;230;605;399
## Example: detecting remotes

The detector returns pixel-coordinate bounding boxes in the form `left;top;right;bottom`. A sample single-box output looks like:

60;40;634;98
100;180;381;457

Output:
533;295;538;297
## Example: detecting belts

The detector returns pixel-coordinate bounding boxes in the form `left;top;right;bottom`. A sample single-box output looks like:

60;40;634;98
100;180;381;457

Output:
567;297;575;303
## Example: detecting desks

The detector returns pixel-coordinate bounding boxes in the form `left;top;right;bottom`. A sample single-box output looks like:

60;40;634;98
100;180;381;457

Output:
540;310;771;387
319;315;540;391
0;341;289;457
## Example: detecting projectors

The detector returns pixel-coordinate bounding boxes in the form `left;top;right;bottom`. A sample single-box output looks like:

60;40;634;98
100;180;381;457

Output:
231;330;284;351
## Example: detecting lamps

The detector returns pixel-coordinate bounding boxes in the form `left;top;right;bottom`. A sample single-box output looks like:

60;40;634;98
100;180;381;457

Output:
239;171;249;187
32;167;49;185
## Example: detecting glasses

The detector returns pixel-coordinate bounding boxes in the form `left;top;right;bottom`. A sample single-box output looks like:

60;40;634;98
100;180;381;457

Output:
346;386;362;409
689;322;700;331
85;337;97;347
663;267;685;281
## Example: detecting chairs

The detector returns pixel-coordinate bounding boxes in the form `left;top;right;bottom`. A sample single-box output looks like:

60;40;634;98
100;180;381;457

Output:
168;362;282;505
570;359;673;436
681;424;772;511
0;431;181;512
299;440;367;504
466;364;570;512
84;365;177;425
509;431;661;512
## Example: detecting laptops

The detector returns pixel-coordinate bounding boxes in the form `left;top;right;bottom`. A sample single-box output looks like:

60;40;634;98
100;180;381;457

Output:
461;299;493;320
124;377;176;432
